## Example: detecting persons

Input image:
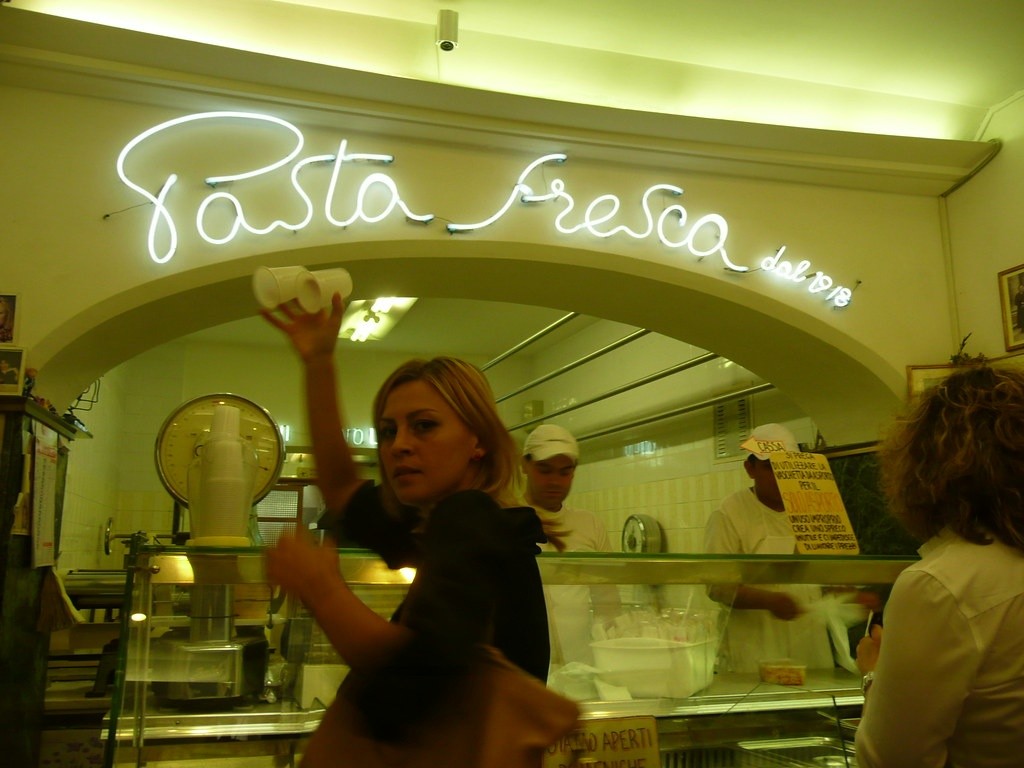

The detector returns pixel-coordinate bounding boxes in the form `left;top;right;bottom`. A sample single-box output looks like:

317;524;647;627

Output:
855;368;1024;768
521;424;623;703
258;290;572;768
704;422;877;675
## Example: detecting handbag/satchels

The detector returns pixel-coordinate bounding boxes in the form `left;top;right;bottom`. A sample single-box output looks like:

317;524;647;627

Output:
297;644;579;767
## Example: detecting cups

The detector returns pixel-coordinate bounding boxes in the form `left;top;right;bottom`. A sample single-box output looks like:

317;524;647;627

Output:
188;405;247;539
296;268;353;314
251;265;310;311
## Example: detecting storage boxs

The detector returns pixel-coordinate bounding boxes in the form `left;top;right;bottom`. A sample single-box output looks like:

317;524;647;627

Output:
592;634;717;697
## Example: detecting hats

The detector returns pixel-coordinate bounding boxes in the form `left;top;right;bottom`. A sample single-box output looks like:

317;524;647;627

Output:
522;424;579;467
751;423;801;461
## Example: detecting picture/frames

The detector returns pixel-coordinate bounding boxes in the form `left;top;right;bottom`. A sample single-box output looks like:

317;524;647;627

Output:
0;346;28;397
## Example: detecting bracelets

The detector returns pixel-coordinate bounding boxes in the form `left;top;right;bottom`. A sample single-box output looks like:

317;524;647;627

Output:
861;672;874;697
